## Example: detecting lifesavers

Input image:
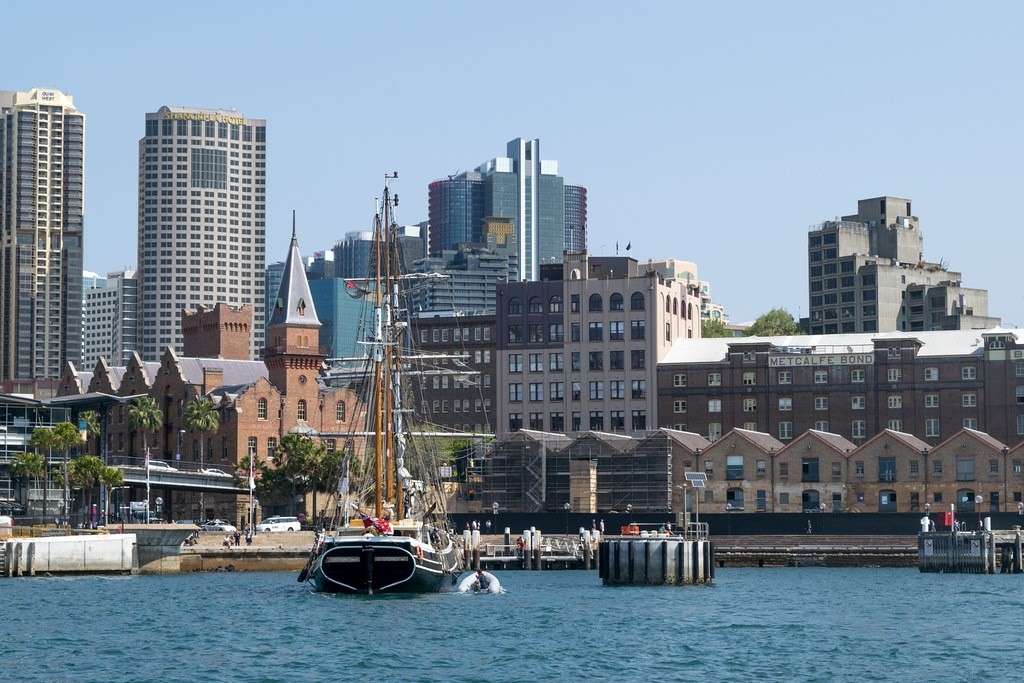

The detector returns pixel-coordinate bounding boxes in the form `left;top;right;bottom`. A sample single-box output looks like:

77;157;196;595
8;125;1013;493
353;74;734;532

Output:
417;545;423;560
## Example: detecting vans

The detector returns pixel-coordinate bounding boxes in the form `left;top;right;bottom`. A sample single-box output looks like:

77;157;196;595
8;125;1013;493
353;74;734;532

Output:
256;516;301;531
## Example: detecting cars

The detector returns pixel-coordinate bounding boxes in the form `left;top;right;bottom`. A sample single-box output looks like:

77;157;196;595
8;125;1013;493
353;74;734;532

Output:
200;519;236;531
0;500;26;516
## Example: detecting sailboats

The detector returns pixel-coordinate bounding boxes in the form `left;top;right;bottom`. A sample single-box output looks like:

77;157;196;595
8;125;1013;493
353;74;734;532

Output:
299;173;469;601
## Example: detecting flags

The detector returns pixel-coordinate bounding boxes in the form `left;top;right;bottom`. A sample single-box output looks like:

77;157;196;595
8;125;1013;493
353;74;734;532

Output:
361;513;391;534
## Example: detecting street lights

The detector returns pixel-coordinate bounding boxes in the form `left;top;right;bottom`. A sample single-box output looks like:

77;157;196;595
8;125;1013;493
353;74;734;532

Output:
683;483;690;538
627;502;635;523
818;502;826;534
492;501;501;535
925;501;930;515
975;494;984;532
177;429;185;468
563;502;571;535
727;504;732;535
107;486;130;524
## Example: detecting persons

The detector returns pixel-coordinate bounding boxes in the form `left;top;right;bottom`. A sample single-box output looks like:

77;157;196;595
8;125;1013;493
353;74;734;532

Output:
805;520;812;534
591;519;596;535
666;520;674;535
931;520;936;532
954;518;959;531
221;531;252;549
579;526;585;539
600;518;605;535
466;520;491;535
476;571;487;589
517;537;524;556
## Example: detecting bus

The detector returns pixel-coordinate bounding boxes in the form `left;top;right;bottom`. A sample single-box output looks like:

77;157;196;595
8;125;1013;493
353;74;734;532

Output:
132;512;155;524
144;518;164;524
